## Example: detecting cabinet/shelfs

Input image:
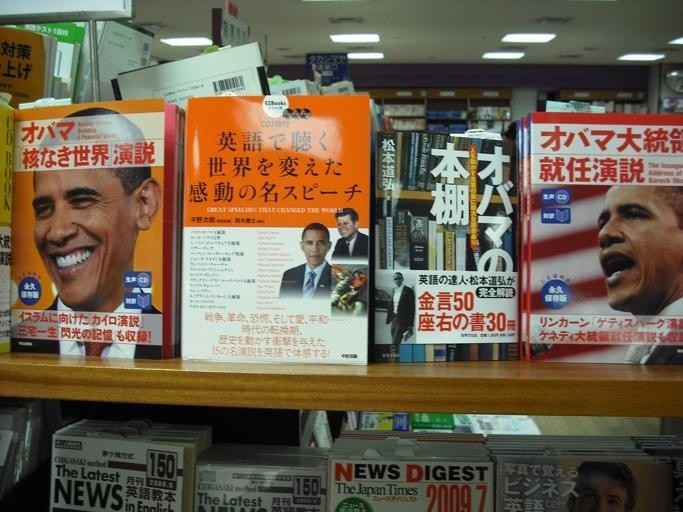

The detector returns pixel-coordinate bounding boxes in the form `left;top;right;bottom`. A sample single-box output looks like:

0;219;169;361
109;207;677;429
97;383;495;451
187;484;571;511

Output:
555;87;647;107
0;351;683;444
355;87;512;134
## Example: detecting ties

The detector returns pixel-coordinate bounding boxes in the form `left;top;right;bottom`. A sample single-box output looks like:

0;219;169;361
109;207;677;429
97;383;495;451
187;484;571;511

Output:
303;272;316;297
83;342;112;358
